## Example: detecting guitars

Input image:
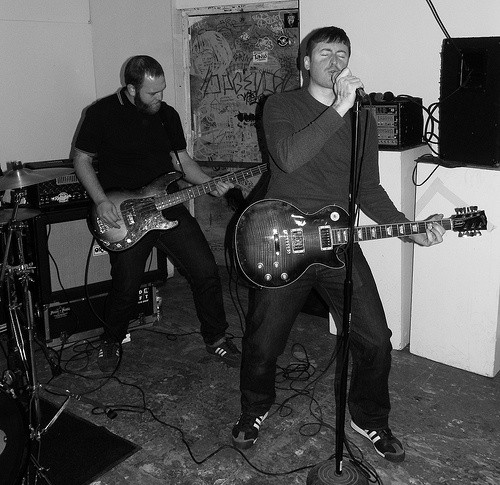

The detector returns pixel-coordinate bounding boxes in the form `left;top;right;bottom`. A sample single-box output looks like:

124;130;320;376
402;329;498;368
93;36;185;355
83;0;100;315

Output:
236;198;487;291
87;163;268;252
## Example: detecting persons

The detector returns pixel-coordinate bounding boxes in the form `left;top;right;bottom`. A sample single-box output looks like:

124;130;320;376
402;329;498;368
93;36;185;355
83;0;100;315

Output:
73;56;242;372
231;26;446;462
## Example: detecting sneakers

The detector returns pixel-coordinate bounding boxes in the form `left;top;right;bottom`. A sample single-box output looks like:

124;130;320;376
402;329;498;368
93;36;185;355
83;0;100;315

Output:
98;340;119;372
231;408;269;451
207;338;242;367
351;417;405;460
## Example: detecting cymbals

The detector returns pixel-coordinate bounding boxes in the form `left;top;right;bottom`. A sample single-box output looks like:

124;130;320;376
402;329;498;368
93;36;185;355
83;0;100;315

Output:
0;207;40;225
0;169;75;191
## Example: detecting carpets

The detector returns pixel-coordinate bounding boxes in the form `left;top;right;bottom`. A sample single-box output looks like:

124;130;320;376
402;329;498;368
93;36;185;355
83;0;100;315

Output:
0;389;144;485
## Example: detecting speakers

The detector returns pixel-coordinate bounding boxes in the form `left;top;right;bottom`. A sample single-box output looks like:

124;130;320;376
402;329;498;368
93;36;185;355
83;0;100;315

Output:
439;37;500;166
33;204;172;305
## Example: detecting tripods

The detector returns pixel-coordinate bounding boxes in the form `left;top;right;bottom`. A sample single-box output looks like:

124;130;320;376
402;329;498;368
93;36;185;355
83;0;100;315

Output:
0;224;117;485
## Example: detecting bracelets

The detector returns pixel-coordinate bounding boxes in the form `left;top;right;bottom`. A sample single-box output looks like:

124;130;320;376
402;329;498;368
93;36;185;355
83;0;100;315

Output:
96;199;105;207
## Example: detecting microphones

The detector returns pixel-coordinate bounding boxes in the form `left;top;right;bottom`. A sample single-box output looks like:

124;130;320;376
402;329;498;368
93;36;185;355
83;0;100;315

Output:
356;88;368;99
